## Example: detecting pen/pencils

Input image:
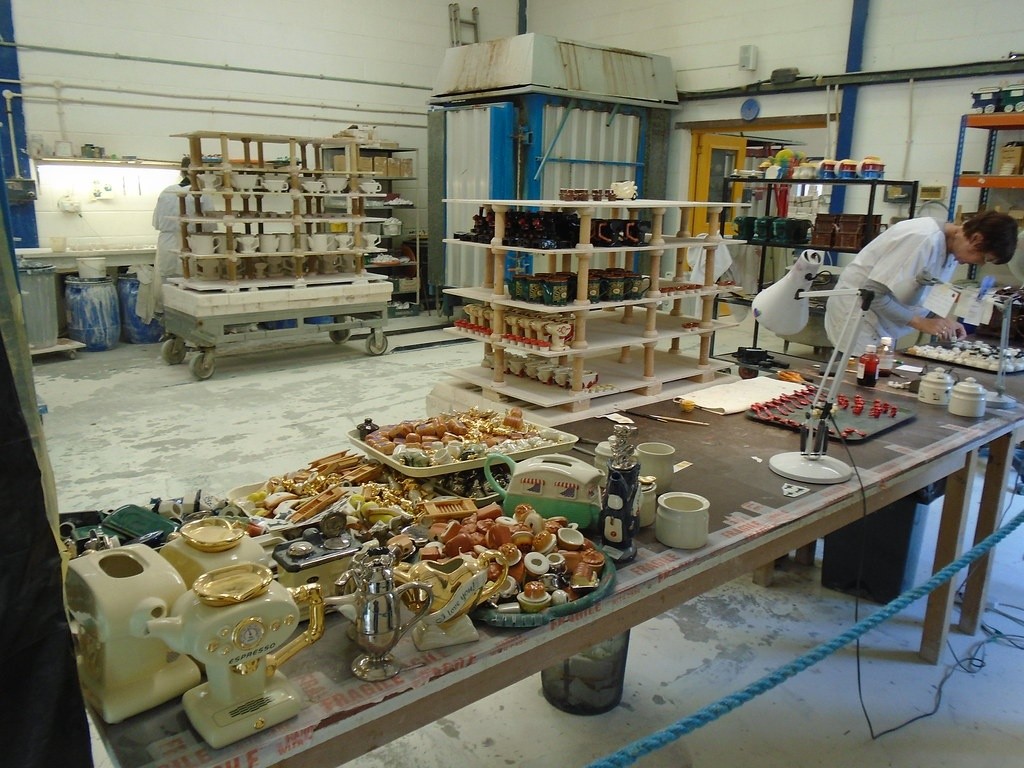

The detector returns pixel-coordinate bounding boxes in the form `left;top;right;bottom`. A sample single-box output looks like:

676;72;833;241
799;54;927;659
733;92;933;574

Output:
892;359;905;365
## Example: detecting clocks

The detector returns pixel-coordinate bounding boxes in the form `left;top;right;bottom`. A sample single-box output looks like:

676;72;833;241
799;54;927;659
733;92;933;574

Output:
740;98;760;122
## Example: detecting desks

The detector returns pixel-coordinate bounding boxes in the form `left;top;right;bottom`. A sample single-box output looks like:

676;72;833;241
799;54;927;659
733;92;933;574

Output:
69;375;1013;768
811;336;1024;635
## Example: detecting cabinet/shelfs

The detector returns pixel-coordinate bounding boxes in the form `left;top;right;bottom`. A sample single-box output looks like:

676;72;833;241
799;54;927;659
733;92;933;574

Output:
428;197;753;423
161;130;394;380
930;113;1024;347
709;178;920;376
322;147;421;320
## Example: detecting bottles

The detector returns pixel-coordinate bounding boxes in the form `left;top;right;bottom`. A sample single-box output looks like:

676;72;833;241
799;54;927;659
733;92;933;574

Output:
876;337;894;378
856;344;881;386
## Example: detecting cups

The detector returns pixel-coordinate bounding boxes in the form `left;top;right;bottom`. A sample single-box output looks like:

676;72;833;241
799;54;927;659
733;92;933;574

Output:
636;442;676;492
50;236;66;252
382;223;402;236
53;140;72;158
504;271;576;307
587;266;652;304
591;218;652;247
432;440;464;464
680;399;695;413
69;242;148;251
81;143;105;158
653;491;710;549
558;529;583;550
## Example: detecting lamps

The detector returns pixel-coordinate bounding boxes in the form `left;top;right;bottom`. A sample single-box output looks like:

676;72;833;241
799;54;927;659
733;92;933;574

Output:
750;250;874;485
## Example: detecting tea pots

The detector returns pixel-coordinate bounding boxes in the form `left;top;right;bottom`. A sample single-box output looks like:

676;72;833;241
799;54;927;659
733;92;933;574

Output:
301;177;350;195
196;173;222;192
358;183;381;195
184;233;221;255
193;253;357;281
293;233;336;252
261;233;292;253
216;235;259;253
261;179;288;193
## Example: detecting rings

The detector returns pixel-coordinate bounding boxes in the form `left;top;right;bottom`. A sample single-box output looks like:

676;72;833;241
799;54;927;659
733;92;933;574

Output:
936;333;938;336
941;331;945;334
942;326;946;329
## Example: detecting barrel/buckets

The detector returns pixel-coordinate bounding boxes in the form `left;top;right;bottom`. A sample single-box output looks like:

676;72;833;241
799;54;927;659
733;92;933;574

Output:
118;275;165;344
260;319;297;330
75;256;108;279
15;259;58;348
303;316;335;324
65;276;120;353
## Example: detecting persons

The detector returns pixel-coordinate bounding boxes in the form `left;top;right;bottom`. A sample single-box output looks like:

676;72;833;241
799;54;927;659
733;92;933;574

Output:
151;155;220;344
823;211;1020;357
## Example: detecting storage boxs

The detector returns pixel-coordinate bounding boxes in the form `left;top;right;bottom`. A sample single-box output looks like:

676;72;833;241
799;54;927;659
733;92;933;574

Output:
373;157;387;177
401;158;413;177
333;155;346;176
359;157;374;176
388;158;401;177
998;146;1023;175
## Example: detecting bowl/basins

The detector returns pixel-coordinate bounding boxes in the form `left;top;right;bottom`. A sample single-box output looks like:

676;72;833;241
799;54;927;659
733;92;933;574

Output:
235;175;259;189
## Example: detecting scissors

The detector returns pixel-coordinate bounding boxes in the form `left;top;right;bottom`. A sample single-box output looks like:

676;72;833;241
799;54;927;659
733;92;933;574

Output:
777;370;830;391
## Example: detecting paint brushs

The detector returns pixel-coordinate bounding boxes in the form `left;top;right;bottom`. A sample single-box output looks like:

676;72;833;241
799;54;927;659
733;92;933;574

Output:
890;372;909;379
614;407;668;423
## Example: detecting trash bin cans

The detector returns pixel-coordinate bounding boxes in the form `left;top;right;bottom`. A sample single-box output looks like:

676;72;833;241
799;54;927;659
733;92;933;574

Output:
542;629;630;714
